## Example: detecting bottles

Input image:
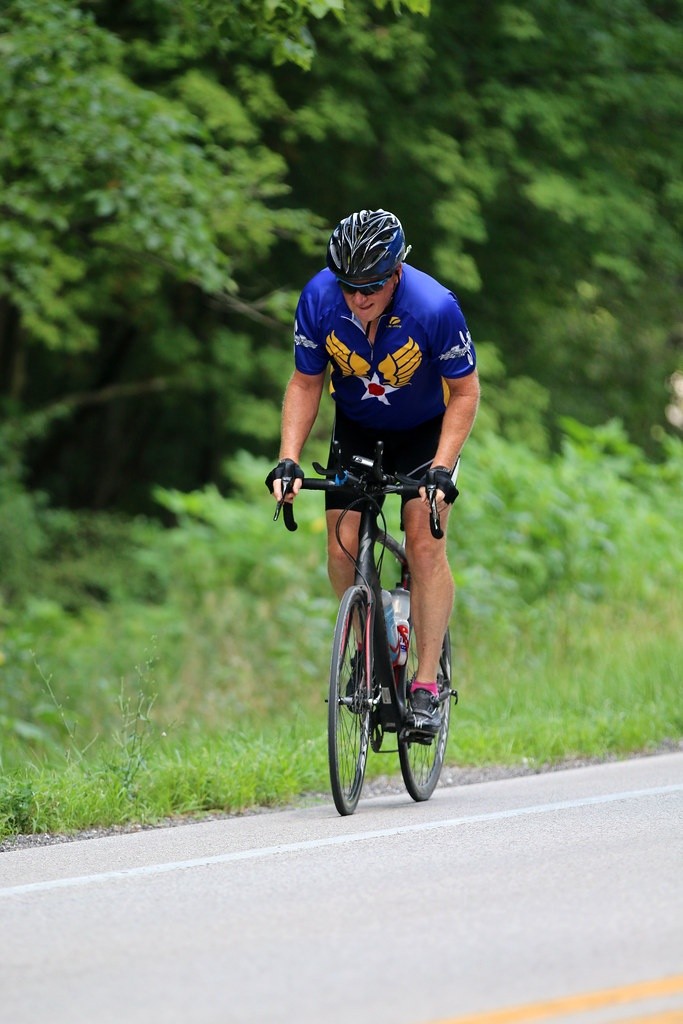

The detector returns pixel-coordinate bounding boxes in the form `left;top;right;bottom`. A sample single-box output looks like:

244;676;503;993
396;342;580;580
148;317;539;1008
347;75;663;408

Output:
390;582;411;665
382;590;399;662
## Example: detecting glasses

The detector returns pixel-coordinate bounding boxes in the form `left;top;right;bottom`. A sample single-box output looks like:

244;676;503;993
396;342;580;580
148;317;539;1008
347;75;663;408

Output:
334;274;393;296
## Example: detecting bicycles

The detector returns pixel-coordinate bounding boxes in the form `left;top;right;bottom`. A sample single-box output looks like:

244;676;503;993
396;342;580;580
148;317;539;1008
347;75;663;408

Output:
274;432;461;816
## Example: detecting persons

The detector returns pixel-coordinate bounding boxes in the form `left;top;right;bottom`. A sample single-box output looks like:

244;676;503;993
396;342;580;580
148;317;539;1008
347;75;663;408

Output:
264;208;480;735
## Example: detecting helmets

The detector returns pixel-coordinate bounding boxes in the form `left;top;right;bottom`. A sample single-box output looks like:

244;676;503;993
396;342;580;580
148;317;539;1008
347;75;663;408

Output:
326;208;406;280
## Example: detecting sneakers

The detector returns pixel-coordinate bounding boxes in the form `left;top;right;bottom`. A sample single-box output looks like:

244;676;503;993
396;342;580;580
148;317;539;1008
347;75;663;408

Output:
345;650;367;713
404;688;440;727
